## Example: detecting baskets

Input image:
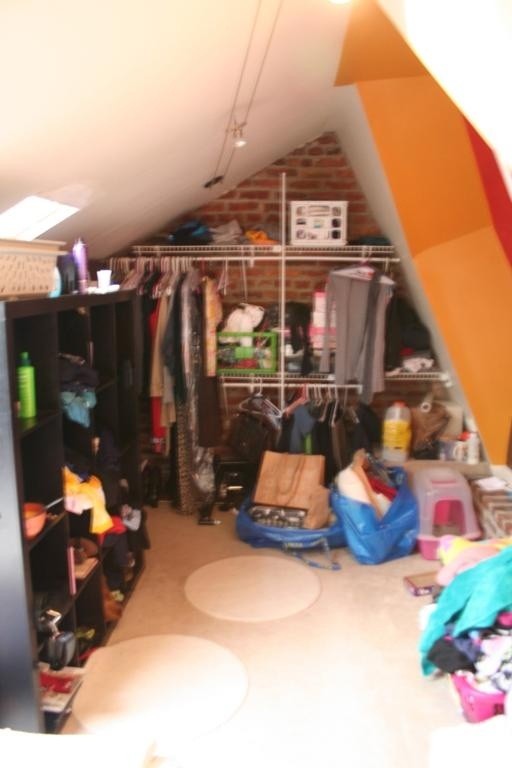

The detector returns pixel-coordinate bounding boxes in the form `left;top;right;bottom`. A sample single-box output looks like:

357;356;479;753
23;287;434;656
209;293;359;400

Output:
0;238;69;302
287;200;349;247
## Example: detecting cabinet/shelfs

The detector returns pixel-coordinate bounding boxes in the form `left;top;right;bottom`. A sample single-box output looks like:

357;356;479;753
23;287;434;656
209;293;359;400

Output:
0;287;148;731
129;243;448;380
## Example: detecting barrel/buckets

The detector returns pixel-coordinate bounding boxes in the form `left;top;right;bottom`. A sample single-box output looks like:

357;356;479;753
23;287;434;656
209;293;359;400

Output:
381;399;412;464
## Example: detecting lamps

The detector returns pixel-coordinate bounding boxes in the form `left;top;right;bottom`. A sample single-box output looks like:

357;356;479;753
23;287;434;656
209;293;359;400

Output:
227;116;250;148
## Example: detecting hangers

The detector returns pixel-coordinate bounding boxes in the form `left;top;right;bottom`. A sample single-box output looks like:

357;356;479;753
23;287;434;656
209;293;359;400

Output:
284;380;365;427
236;377;283;418
332;244;396;299
108;254;248;299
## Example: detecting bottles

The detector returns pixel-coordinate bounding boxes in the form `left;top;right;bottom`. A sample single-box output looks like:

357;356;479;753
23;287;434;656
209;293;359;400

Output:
72;238;90;294
467;431;482;464
15;352;36;417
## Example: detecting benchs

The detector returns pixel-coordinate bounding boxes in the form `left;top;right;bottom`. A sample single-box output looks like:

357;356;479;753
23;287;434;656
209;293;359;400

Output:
466;472;512;544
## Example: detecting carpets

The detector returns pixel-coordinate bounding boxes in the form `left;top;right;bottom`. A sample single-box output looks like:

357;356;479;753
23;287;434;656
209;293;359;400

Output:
183;553;323;622
71;631;247;732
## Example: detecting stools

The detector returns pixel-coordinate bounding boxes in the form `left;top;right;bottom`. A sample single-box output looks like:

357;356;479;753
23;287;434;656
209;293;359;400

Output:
411;463;482;559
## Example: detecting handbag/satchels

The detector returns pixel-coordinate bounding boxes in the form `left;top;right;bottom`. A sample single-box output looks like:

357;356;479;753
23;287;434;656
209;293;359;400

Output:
228;392;273;464
168;221;211;245
251;450;326;511
331;463;419;565
237;494;346;570
412;403;448;454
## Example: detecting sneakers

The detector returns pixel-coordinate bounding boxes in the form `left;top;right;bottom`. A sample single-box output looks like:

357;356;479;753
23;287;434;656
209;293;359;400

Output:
71;537;98;563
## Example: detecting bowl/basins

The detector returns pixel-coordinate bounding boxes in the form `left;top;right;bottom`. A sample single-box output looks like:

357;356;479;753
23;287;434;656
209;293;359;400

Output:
18;500;49;542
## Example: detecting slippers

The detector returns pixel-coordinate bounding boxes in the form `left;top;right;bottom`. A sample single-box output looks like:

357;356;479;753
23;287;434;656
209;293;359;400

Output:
246;231;277;244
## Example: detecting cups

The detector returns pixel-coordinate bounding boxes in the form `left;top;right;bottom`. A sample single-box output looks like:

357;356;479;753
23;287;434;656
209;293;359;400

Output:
96;268;112;288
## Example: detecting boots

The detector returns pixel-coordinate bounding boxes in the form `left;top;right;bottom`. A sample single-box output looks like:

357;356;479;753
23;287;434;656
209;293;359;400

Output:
144;470;159;507
220;485;244;511
